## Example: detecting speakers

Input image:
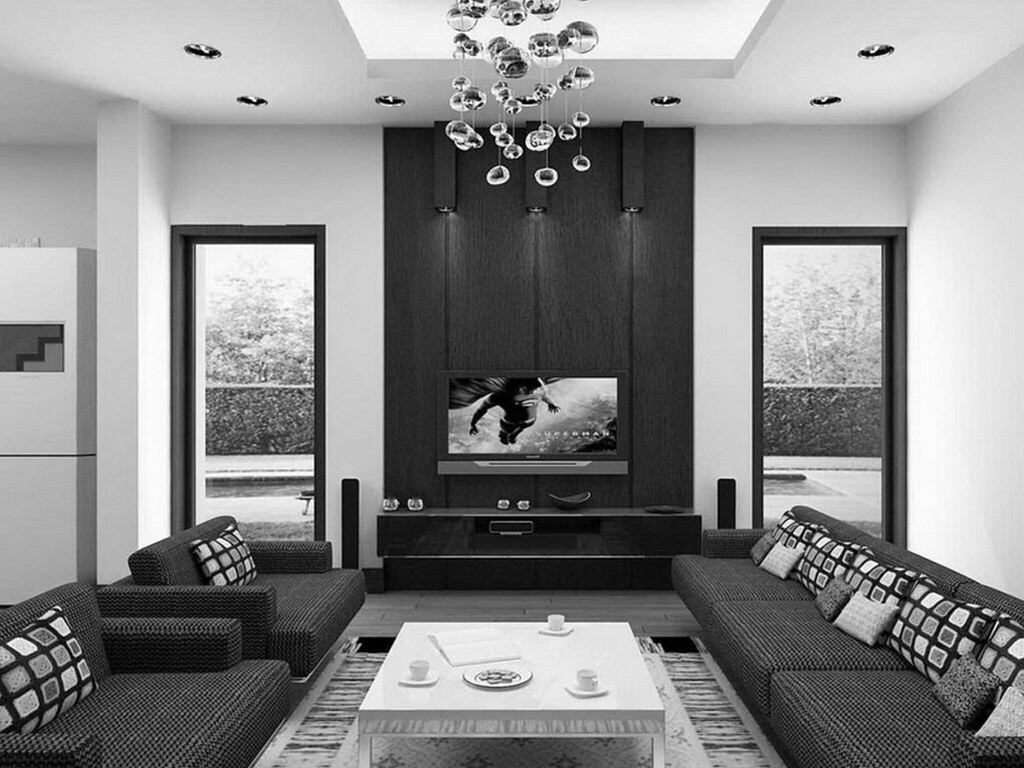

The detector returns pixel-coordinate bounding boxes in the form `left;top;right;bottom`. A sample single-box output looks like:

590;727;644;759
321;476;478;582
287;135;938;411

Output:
340;478;359;570
717;479;737;530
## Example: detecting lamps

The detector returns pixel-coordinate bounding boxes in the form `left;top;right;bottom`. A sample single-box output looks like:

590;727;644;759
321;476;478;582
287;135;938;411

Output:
432;122;458;214
620;120;645;213
525;120;550;213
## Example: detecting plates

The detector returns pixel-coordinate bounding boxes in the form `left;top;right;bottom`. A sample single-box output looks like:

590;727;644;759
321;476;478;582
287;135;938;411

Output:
463;662;533;689
540;624;573;636
399;673;440;685
547;492;591;510
565;678;607;697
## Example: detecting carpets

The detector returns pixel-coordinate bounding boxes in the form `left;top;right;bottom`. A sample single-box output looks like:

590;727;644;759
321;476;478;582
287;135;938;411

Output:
248;635;788;768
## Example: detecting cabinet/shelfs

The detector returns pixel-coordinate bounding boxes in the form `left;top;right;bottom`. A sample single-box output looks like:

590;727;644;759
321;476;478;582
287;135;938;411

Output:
376;505;702;590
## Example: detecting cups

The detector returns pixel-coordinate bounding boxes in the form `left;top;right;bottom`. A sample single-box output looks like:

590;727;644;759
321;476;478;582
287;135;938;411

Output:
577;668;598;690
547;614;564;630
409;660;429;680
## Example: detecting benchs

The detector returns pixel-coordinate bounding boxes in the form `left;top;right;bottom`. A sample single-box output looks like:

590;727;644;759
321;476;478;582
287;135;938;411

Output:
94;515;367;683
673;505;1024;768
0;580;296;768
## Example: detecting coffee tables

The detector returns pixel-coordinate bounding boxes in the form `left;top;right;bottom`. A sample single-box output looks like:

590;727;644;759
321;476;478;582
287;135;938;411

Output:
358;622;667;767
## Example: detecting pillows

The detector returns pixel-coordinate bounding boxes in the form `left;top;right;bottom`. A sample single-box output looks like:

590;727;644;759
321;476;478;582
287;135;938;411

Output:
750;510;1024;737
190;522;259;587
0;603;99;736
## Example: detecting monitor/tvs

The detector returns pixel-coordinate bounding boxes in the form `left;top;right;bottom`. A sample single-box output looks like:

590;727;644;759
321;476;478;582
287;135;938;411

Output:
434;369;629;476
